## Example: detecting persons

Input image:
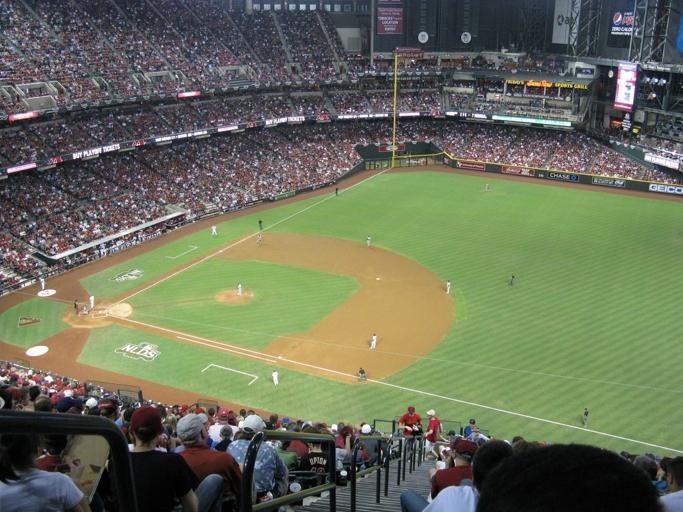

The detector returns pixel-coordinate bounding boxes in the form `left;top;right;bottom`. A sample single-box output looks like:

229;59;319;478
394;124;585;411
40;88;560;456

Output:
1;1;683;512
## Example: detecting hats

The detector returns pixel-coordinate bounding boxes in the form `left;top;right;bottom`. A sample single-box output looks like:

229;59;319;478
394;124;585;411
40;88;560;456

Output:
450;439;477;454
426;409;436;417
408;406;415;413
446;430;456;436
1;359;164;443
165;402;372;442
